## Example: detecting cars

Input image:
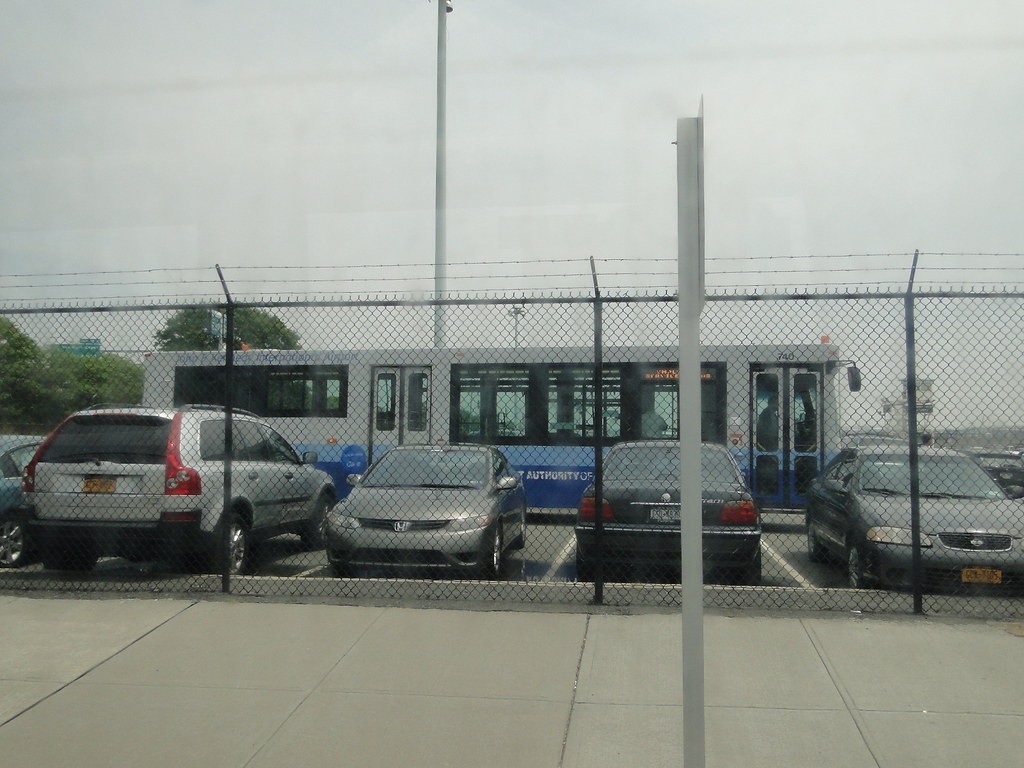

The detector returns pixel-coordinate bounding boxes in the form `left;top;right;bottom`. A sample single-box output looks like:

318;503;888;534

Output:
0;435;49;567
322;446;526;580
574;440;762;588
805;446;1024;598
957;448;1024;499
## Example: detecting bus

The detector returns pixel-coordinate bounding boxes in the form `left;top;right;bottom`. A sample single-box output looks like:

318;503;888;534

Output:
142;346;861;526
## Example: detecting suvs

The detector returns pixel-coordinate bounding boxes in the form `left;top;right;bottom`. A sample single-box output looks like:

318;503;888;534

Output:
22;406;337;578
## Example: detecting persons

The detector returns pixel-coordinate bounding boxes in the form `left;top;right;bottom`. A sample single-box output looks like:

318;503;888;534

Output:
758;396;778;443
641;411;668;438
